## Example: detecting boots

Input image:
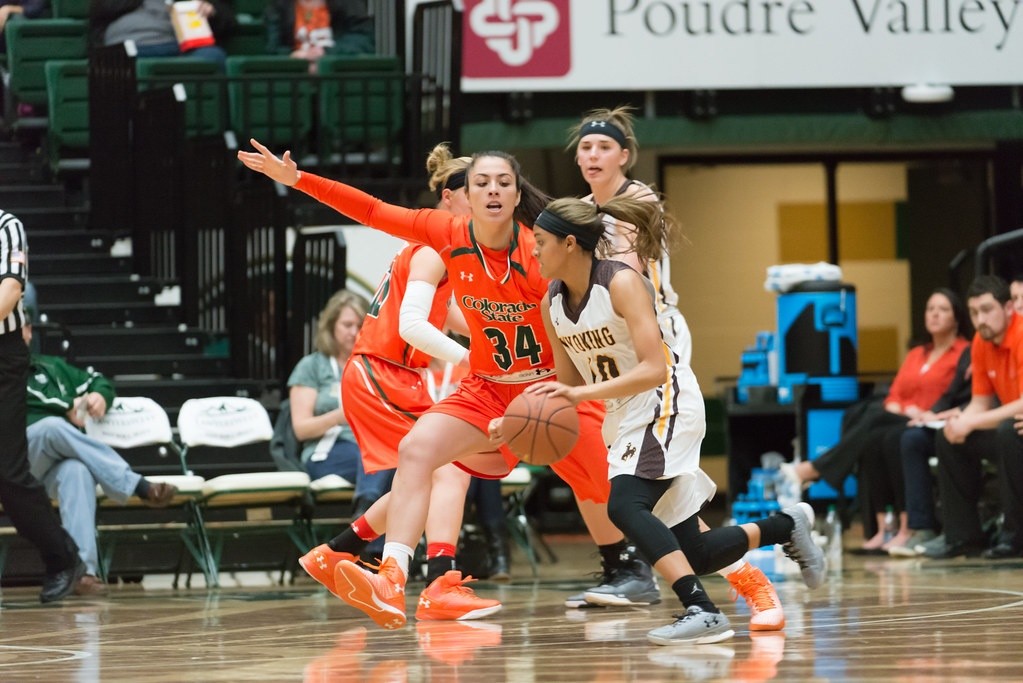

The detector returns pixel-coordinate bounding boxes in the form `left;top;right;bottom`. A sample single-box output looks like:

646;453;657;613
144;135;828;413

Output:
486;521;512;580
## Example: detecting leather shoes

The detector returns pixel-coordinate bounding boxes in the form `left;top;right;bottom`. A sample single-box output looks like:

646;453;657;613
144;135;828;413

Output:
40;561;87;603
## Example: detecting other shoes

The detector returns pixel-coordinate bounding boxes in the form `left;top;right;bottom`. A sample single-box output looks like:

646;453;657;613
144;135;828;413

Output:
140;483;179;506
779;462;802;483
849;527;1016;560
76;575;108;596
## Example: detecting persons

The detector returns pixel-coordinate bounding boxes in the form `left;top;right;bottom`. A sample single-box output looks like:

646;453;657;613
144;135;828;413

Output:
488;198;827;645
286;290;396;564
237;139;786;631
569;105;680;607
0;0;235;73
781;274;1023;559
297;142;504;621
0;206;89;604
22;306;179;596
464;476;511;578
275;0;347;74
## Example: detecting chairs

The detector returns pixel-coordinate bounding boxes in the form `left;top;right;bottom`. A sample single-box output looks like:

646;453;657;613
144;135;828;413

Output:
0;391;542;599
0;0;405;173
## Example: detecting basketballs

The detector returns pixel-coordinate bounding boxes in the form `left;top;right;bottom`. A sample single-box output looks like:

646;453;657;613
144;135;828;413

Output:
502;390;580;466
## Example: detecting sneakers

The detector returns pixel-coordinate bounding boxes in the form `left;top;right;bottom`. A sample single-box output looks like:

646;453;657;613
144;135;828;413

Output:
334;557;407;630
299;544;360;597
776;502;827;590
415;621;503;666
726;562;786;631
583;551;661;606
646;606;733;646
414;570;502;621
564;561;607;606
647;645;735;678
